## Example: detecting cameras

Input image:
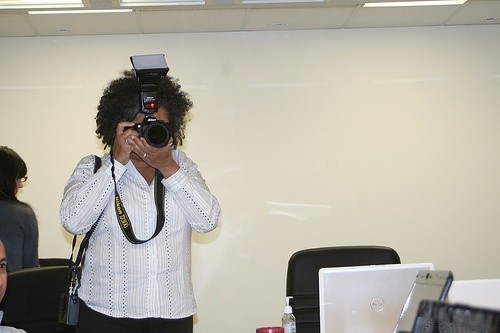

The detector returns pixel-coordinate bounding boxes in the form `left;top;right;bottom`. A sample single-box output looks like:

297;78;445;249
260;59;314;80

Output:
125;53;174;149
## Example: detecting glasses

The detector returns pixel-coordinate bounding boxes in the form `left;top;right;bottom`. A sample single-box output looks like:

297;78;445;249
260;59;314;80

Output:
21;176;27;182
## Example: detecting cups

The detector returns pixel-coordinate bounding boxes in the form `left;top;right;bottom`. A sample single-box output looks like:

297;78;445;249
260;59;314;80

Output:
255;326;284;333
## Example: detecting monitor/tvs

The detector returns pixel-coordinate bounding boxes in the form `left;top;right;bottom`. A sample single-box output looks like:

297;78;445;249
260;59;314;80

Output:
316;261;435;333
394;267;451;332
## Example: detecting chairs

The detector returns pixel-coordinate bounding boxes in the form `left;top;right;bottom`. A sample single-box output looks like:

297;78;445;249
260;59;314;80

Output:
0;259;82;333
286;246;401;333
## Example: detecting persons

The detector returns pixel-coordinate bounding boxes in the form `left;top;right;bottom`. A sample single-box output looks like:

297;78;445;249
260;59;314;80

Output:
60;69;222;333
0;239;27;333
0;146;40;272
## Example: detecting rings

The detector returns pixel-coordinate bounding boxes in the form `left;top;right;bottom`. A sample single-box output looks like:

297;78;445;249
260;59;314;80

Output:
125;138;128;143
143;152;147;158
